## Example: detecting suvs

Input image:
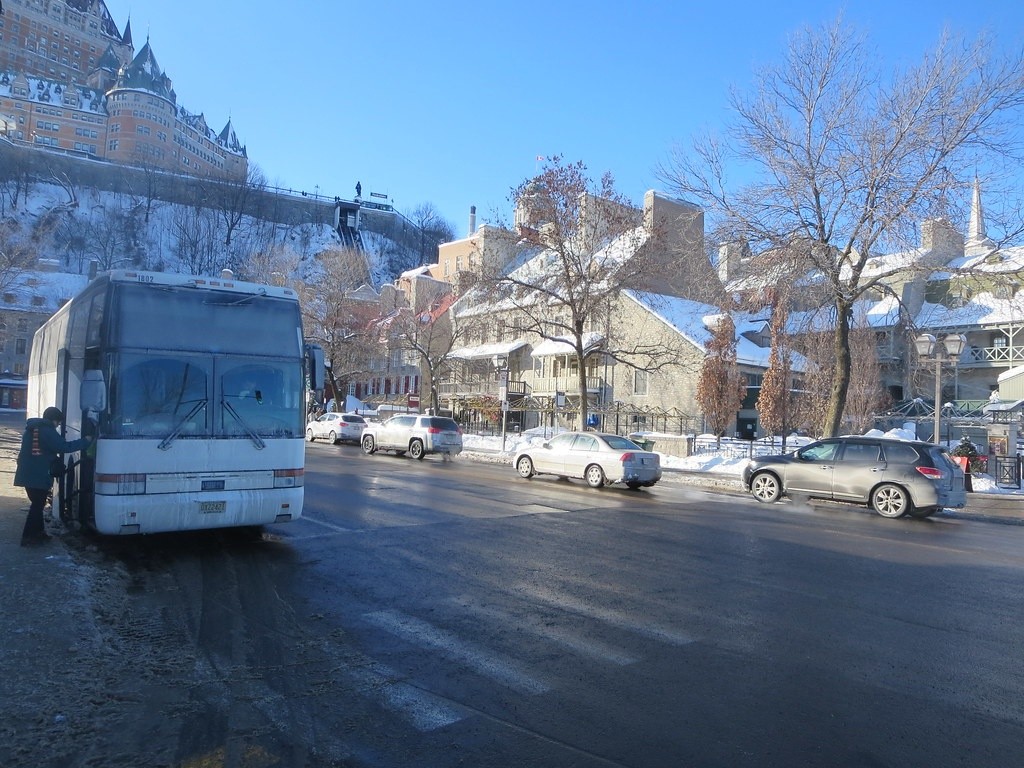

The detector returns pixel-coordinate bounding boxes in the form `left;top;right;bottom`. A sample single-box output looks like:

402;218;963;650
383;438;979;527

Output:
362;415;463;461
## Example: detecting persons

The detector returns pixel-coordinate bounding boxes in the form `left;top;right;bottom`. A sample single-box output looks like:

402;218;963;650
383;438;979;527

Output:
308;408;326;422
14;406;92;539
230;375;259;409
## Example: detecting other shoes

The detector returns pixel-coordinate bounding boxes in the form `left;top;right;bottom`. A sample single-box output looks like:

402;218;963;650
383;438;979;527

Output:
21;537;43;548
33;532;52;542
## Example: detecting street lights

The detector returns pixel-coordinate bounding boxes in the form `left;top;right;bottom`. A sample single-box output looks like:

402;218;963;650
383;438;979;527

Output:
914;333;967;443
492;354;509;449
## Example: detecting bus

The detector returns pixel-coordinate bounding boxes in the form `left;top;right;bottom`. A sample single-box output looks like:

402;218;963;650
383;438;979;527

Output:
24;267;328;539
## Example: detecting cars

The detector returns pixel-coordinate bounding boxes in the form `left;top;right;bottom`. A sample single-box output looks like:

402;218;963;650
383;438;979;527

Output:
512;431;662;490
742;435;968;519
307;409;367;445
